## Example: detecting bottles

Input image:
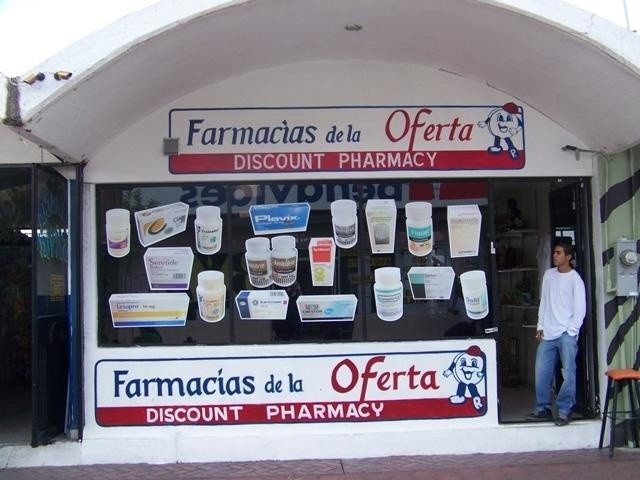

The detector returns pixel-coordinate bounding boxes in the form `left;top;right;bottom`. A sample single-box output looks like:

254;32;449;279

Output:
244;235;297;289
403;201;435;258
194;205;223;257
195;271;228;323
373;266;404;322
460;269;491;321
329;199;358;251
104;209;133;258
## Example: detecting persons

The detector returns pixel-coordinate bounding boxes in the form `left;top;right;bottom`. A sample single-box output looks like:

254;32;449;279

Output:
526;242;586;426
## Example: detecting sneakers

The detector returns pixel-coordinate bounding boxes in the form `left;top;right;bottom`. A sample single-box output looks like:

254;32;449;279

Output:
556;417;568;426
526;407;553;422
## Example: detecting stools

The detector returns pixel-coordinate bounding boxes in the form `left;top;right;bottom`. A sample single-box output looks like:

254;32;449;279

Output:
597;368;640;459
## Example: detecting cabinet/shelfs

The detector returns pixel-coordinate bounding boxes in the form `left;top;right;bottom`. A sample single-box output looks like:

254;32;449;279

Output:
495;227;540;328
